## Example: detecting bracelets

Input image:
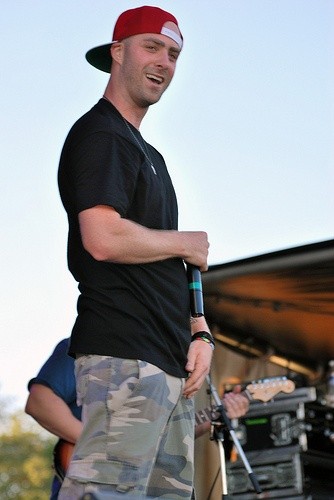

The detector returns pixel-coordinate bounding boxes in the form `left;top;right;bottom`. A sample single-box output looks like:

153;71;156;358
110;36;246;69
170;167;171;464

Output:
191;331;217;351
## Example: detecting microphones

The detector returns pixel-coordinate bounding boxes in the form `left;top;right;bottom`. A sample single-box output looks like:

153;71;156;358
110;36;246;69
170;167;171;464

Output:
186;263;204;317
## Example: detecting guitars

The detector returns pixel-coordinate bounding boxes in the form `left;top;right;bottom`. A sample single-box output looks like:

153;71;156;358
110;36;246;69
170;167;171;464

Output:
52;375;295;482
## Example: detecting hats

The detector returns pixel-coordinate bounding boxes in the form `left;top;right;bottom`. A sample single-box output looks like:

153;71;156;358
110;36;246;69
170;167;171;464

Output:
85;6;183;74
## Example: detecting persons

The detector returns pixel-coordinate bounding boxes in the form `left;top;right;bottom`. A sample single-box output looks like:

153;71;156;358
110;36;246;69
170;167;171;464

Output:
23;336;253;500
57;7;216;500
